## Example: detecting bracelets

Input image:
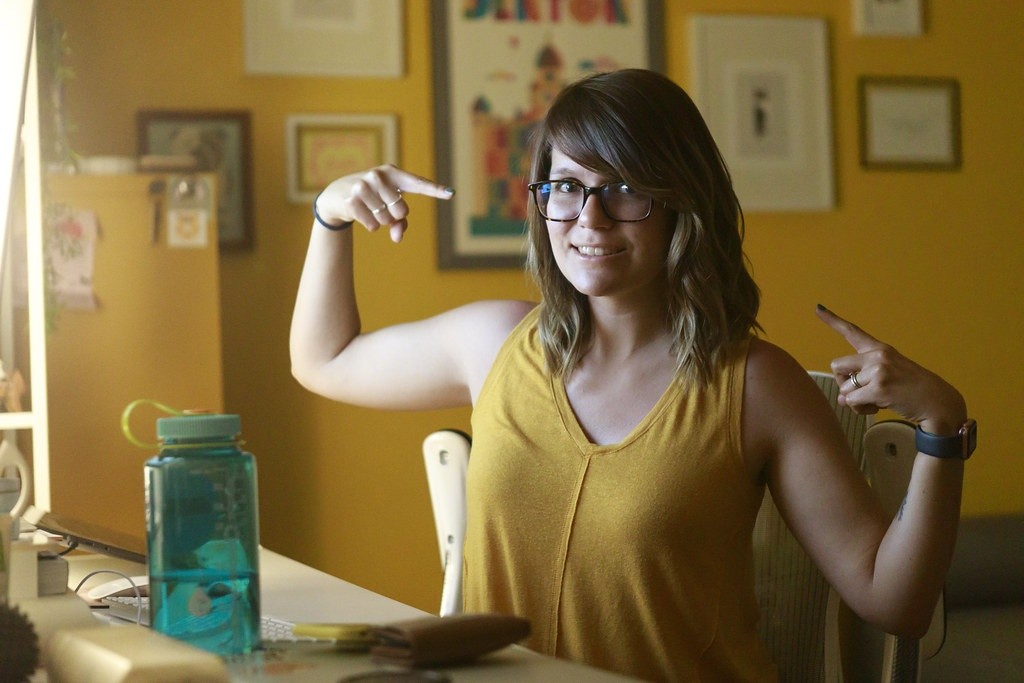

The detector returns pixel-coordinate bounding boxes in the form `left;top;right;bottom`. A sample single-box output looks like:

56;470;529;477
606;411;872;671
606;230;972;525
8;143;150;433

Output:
312;190;357;232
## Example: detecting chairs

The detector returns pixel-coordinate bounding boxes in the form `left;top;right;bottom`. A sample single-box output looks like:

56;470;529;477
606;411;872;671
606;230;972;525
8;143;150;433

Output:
422;370;946;683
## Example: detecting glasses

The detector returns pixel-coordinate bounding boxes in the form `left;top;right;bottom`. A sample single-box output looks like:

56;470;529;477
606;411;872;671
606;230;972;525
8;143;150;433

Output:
528;178;656;223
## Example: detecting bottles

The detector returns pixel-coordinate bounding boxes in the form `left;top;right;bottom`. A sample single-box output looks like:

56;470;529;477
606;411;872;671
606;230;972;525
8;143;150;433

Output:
122;398;261;659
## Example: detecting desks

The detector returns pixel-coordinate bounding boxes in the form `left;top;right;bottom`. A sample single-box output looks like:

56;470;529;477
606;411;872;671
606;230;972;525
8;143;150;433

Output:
0;519;647;683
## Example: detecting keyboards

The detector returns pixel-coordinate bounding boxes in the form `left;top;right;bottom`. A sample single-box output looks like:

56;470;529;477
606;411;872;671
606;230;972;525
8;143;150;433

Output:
103;598;337;648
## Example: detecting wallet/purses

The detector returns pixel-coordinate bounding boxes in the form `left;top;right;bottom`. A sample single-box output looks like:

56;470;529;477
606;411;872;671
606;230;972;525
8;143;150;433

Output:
369;606;531;667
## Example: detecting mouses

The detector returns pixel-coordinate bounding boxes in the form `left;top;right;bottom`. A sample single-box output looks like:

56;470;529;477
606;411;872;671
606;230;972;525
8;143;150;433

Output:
88;576;147;602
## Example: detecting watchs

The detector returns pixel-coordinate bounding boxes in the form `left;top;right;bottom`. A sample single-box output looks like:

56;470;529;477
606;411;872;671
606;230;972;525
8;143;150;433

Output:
915;419;979;460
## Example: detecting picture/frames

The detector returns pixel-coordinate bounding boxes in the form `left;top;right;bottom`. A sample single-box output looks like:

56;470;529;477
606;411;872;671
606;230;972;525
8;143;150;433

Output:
857;74;962;173
854;0;926;40
244;0;406;79
137;111;256;252
287;113;400;203
690;14;836;213
429;0;666;273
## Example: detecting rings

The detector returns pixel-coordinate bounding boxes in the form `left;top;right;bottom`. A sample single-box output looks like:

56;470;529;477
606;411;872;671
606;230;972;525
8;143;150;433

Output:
849;372;862;388
387;188;403;208
371;205;387;216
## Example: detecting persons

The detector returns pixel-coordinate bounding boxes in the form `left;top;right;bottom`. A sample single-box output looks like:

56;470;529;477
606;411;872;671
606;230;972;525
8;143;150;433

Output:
285;70;968;683
